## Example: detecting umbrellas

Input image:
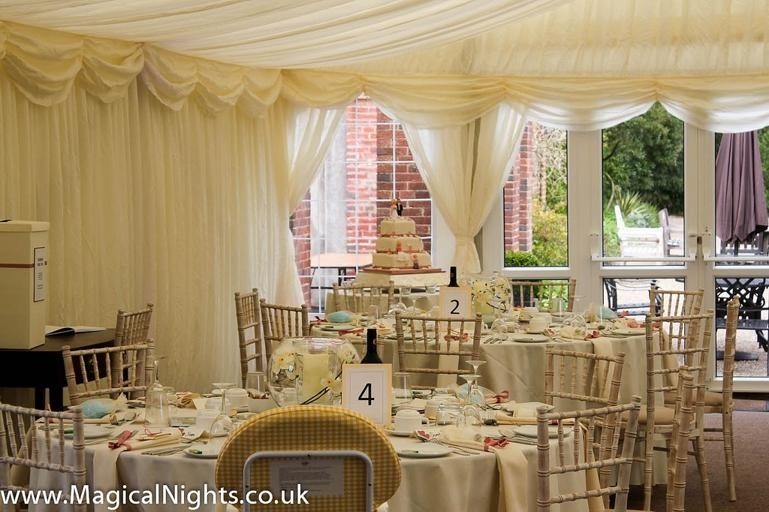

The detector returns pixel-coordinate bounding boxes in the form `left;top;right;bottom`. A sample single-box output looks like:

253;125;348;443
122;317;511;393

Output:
716;128;769;259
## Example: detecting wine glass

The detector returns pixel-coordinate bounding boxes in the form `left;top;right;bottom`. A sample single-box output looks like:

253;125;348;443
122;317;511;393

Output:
458;360;486;432
209;383;234;455
144;354;170;426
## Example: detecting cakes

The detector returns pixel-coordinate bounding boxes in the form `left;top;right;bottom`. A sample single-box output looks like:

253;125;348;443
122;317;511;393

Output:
373;198;432;268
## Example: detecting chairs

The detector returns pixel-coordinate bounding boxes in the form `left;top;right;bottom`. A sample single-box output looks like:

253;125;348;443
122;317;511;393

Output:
0;206;769;512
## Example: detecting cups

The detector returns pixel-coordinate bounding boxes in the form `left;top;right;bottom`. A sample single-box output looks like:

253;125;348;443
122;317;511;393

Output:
246;372;265;411
224;389;248;412
395;410;429;430
392;372;412;409
435;403;463;444
205;397;230;416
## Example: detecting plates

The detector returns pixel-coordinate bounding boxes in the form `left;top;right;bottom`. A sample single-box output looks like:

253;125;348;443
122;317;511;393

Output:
60;424;112;439
184;439;224;458
396;442;454;457
613;328;645;335
319;324;353;331
512;334;548;342
384;427;422;436
395;389;431;398
514;424;571;437
503;402;555;413
